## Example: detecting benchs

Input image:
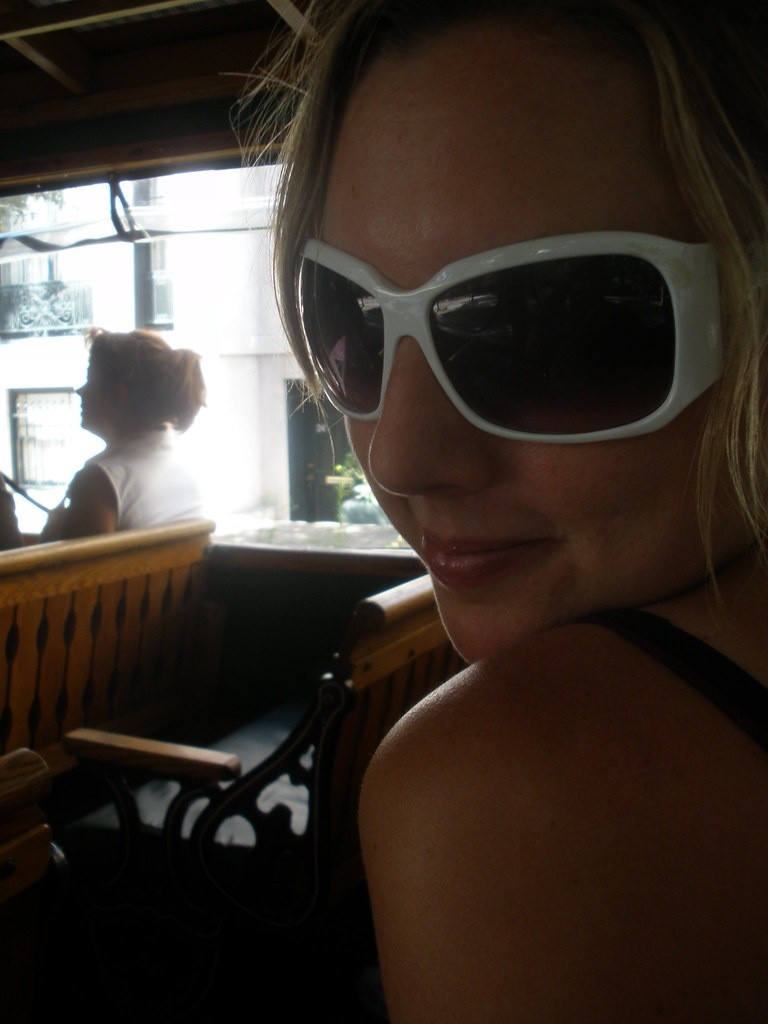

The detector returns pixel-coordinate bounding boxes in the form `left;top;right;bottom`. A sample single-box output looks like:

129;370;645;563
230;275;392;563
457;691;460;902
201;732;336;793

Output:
63;567;470;1024
0;528;211;846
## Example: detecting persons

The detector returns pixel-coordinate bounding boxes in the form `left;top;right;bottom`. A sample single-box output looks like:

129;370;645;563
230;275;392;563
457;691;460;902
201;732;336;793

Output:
0;328;207;874
278;0;768;1024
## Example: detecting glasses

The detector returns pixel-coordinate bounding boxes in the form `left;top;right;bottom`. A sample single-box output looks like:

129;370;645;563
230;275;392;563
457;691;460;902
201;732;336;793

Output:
294;228;768;445
